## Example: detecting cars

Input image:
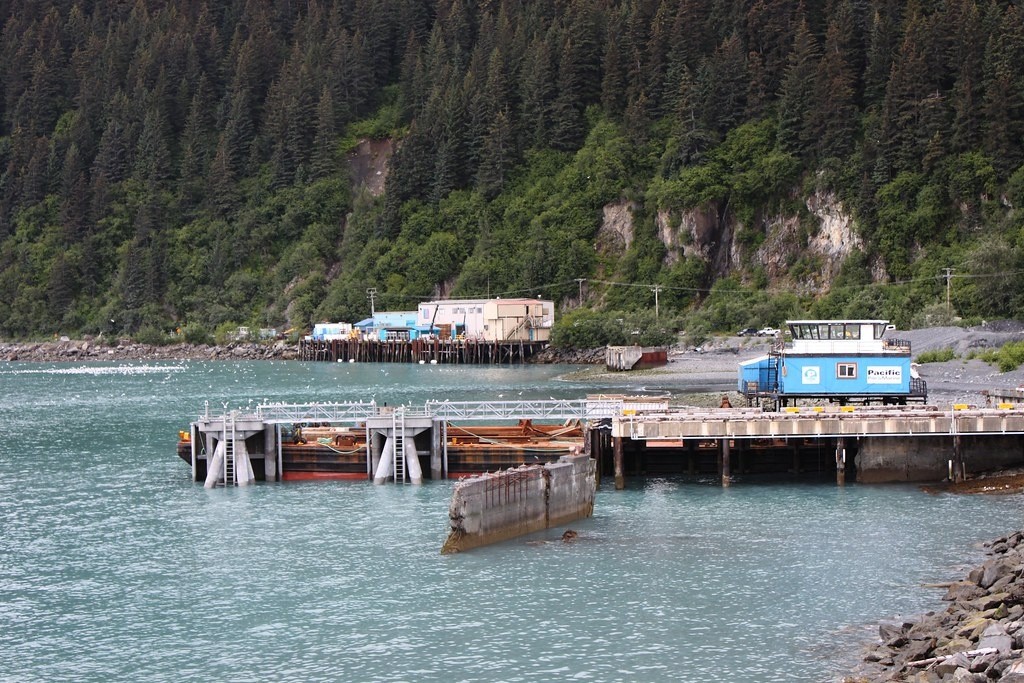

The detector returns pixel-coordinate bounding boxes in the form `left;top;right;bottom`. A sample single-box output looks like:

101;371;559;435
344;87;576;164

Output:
737;328;758;337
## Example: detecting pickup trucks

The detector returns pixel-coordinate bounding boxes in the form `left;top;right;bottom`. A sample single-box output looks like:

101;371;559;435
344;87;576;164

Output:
757;327;780;337
